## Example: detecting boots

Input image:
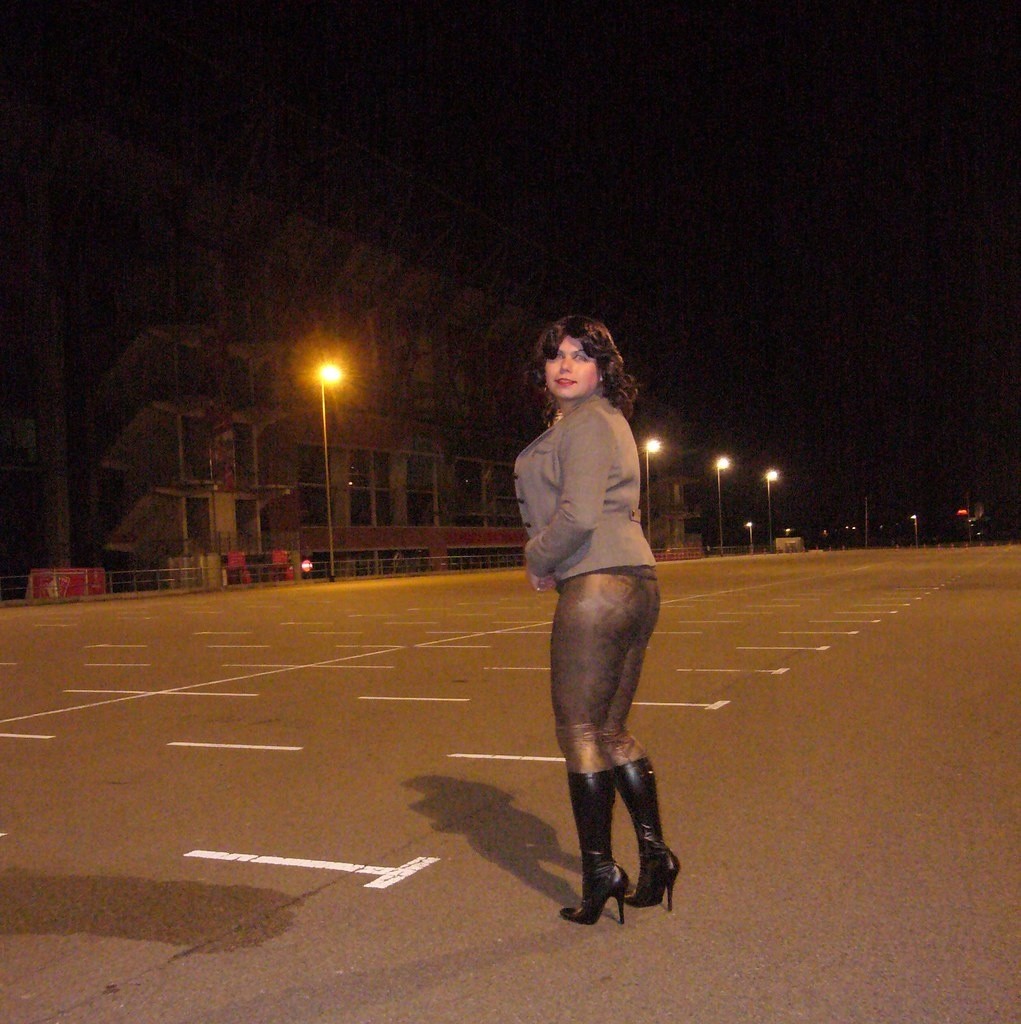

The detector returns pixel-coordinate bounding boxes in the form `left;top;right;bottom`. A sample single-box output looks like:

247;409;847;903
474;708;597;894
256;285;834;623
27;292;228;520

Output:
560;767;630;926
614;756;681;912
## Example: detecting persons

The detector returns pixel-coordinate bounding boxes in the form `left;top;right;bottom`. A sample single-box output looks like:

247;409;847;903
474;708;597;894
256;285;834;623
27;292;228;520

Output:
513;315;681;927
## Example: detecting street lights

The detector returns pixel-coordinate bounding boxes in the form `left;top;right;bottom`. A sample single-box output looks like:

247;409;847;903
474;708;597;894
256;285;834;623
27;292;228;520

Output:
317;362;339;581
715;458;730;557
745;523;754;555
766;470;777;554
909;515;919;549
645;440;662;548
